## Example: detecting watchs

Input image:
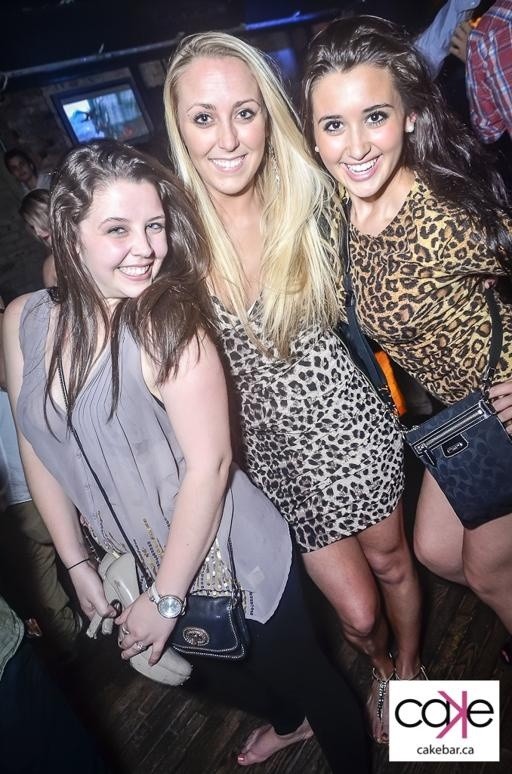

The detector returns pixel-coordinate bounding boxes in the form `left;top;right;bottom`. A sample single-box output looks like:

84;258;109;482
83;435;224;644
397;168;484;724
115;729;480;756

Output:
145;583;189;618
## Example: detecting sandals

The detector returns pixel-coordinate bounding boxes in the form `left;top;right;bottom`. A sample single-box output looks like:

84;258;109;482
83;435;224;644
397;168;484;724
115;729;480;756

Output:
194;633;430;764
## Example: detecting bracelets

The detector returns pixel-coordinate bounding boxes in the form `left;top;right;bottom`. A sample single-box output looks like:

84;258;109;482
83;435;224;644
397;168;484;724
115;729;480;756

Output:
66;552;92;575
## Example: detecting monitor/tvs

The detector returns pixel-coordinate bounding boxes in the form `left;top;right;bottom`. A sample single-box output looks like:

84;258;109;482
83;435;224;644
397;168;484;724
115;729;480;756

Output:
51;77;154;147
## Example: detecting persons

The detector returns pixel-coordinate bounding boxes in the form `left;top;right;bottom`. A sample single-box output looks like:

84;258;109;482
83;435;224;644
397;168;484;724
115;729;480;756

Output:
163;32;443;745
6;145;54;194
1;598;47;771
451;1;511;151
20;188;61;285
296;14;512;635
2;141;385;774
410;0;481;83
0;388;82;644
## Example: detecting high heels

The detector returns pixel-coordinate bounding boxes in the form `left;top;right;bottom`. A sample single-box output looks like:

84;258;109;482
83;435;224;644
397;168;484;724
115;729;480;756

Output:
85;552;192;686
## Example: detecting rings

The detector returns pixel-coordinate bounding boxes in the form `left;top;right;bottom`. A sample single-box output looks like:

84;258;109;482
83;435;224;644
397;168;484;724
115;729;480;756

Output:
120;626;130;634
137;640;145;650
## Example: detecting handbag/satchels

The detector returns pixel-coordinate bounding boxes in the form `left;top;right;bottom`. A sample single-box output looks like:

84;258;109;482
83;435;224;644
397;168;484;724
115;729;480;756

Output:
404;384;511;530
168;594;253;663
